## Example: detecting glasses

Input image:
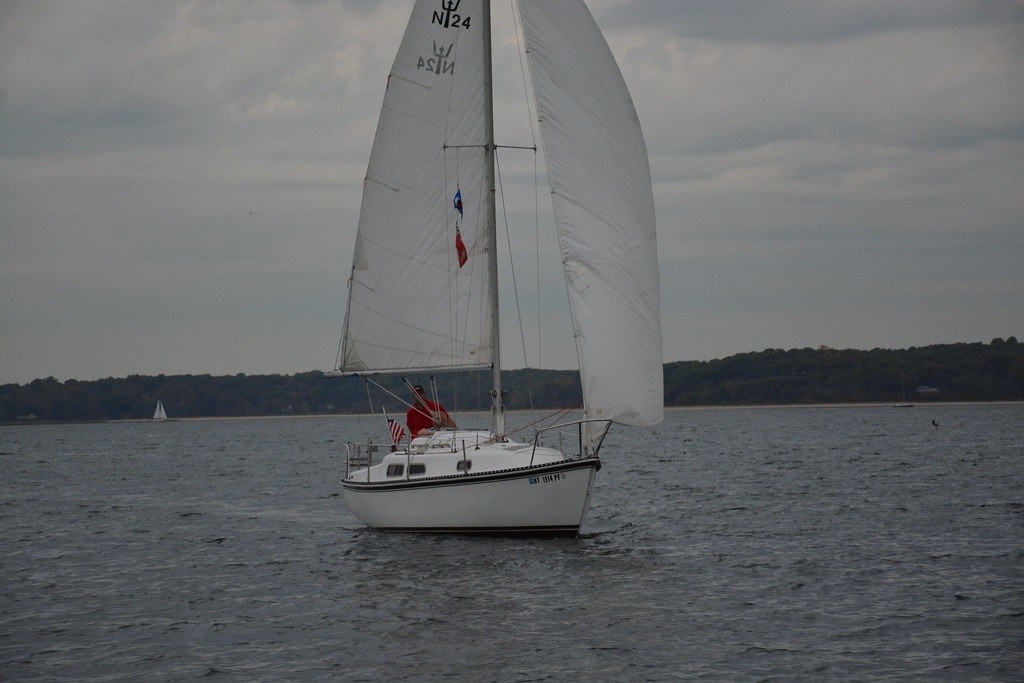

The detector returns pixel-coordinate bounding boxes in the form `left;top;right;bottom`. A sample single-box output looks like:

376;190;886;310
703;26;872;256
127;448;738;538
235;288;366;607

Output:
412;389;421;396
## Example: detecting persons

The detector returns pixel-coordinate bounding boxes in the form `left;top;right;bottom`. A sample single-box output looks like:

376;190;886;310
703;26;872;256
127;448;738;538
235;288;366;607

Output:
406;385;457;436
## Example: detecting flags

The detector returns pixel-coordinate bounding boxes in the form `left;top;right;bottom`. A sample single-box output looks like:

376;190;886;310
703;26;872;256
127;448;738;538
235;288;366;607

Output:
453;190;463;216
382;406;405;445
455;224;468;268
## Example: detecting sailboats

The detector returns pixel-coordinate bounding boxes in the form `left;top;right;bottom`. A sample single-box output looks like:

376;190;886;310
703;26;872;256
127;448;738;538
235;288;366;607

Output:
152;400;167;422
334;0;664;537
